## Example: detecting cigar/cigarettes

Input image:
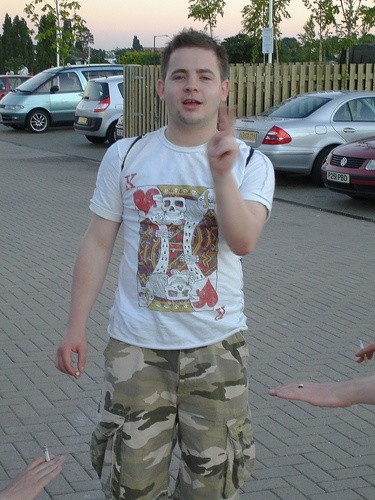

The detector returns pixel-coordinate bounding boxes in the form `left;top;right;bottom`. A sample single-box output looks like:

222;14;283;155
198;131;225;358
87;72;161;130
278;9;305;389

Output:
359;340;370;364
75;369;81;377
44;447;51;462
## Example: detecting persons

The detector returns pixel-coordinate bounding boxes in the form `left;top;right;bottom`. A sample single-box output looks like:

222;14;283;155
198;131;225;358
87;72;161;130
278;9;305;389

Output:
0;454;63;500
49;63;58;86
54;25;276;500
268;345;375;407
17;63;34;90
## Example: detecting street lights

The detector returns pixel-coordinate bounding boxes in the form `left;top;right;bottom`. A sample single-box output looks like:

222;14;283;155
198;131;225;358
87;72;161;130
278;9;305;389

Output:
153;34;169;64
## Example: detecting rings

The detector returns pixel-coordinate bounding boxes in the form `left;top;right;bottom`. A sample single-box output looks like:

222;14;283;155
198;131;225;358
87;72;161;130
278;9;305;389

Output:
298;383;304;388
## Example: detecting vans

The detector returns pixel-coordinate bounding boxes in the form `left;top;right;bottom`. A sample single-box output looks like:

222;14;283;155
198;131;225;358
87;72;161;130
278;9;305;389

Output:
0;64;142;133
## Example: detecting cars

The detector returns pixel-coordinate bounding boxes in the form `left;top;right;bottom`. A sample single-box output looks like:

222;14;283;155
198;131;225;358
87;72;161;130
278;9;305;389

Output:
233;90;375;188
0;75;48;107
114;113;158;141
73;73;155;144
321;136;375;199
